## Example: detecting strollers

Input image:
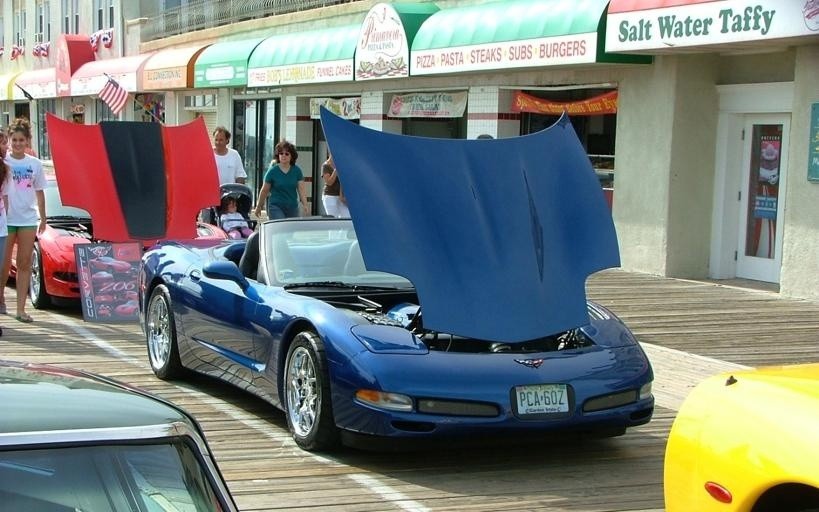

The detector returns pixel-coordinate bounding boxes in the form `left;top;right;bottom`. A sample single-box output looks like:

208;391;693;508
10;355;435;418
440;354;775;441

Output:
211;182;257;237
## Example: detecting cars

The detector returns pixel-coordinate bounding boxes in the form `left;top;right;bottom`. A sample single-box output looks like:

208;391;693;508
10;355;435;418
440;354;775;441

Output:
0;359;237;512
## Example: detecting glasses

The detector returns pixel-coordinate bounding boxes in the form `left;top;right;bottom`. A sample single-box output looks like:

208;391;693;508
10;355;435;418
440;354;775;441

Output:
280;152;290;156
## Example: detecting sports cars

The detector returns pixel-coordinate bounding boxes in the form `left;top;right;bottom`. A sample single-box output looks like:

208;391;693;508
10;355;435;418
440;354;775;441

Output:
7;168;228;307
138;105;655;452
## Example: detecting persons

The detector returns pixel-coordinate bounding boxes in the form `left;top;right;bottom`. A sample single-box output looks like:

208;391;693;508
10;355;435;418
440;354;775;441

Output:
254;140;311;223
200;126;252;228
217;197;254;241
0;116;47;335
321;154;351;219
338;181;347;205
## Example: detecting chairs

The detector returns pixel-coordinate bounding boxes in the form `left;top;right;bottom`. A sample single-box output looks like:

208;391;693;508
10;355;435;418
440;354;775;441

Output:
344;242;366;275
238;231;290;283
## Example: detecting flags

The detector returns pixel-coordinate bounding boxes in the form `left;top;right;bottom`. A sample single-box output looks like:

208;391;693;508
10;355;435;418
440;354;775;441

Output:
97;77;129;117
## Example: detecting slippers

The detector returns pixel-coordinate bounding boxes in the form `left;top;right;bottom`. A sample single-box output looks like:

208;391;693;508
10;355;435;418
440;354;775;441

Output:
15;314;34;323
